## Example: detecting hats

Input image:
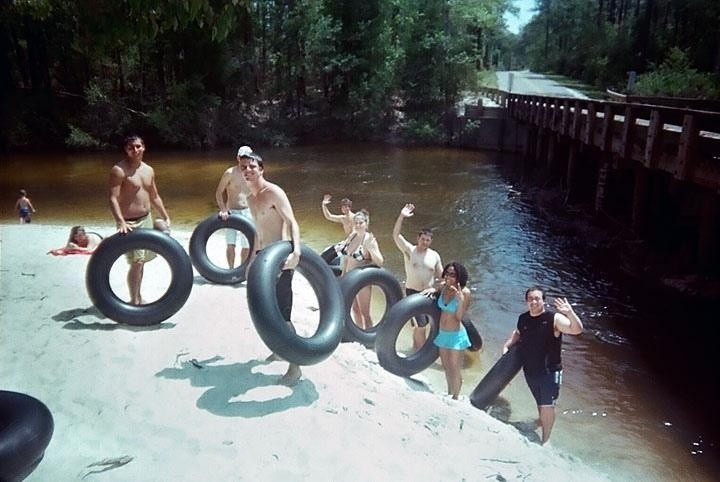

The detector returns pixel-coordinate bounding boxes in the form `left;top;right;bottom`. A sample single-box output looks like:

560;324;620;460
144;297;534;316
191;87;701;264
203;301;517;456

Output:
238;146;252;157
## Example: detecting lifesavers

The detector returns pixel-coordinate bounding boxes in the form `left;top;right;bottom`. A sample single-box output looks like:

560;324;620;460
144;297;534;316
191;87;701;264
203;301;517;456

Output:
189;214;256;284
0;390;54;482
340;265;403;349
461;315;482;352
321;241;363;277
469;342;523;410
247;240;345;365
376;293;440;376
86;228;193;325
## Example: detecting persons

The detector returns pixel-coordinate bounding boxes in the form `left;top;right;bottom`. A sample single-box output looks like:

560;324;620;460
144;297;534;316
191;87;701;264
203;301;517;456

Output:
503;285;584;446
215;145;254;269
109;132;169;305
239;149;303;387
342;208;384;330
47;225;99;256
392;203;444;352
14;187;37;224
419;261;472;400
322;191;357;273
